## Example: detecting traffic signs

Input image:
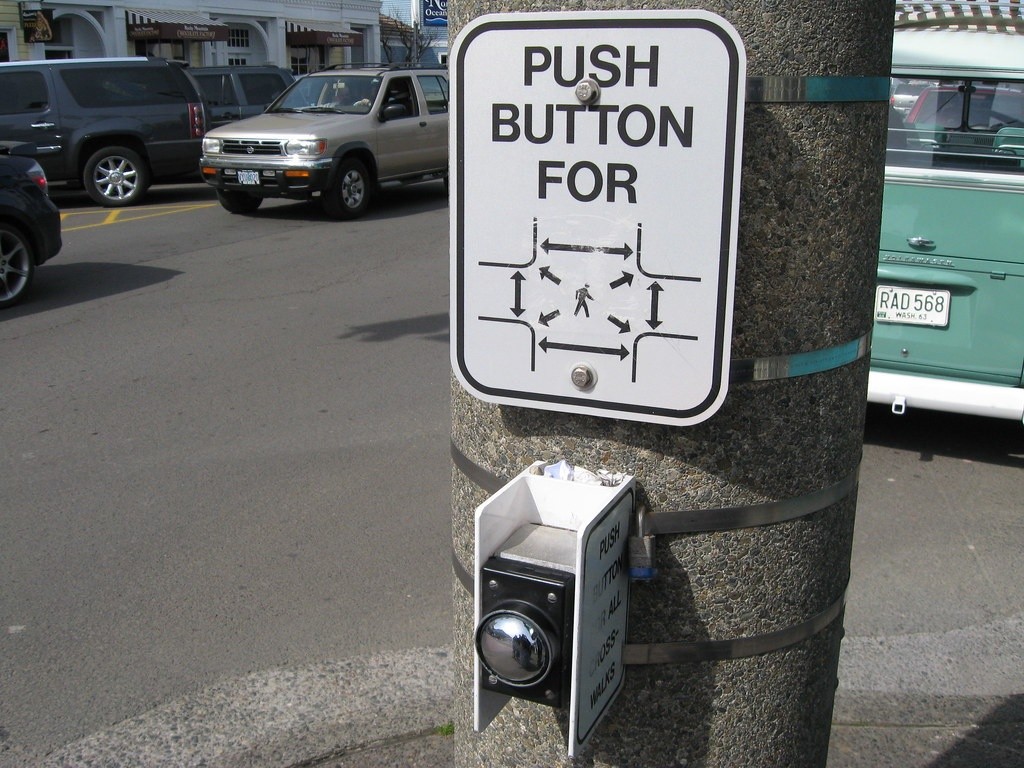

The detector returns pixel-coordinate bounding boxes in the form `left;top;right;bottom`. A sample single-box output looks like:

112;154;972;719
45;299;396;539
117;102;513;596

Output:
446;8;749;426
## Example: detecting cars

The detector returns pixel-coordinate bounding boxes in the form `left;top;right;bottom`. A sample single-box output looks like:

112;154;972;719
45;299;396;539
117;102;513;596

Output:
0;140;63;309
182;65;306;129
904;84;1024;131
0;57;212;208
891;81;930;115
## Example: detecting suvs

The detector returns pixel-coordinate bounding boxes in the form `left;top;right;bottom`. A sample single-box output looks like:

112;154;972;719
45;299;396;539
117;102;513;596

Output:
200;63;448;222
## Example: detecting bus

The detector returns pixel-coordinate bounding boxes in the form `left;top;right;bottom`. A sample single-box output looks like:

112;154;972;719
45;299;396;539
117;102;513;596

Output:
866;27;1024;424
